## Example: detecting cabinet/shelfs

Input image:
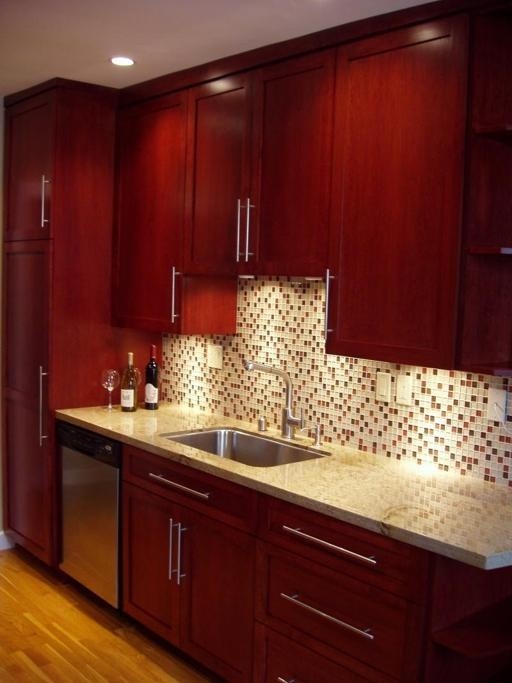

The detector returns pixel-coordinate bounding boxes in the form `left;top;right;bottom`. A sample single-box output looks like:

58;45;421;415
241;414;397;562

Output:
252;492;512;683
186;47;336;276
121;443;261;683
4;77;117;240
326;0;512;374
0;240;163;569
110;87;238;335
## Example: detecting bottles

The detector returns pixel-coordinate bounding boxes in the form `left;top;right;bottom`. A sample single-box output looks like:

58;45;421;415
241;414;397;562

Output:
144;343;160;411
119;351;136;414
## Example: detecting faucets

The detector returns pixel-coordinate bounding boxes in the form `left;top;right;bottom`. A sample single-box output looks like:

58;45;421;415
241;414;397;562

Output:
241;360;305;438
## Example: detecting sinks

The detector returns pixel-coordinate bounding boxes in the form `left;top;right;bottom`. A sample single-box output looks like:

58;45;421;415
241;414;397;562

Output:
163;426;332;469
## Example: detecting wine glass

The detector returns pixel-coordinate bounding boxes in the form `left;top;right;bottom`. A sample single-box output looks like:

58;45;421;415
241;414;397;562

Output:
101;367;120;410
